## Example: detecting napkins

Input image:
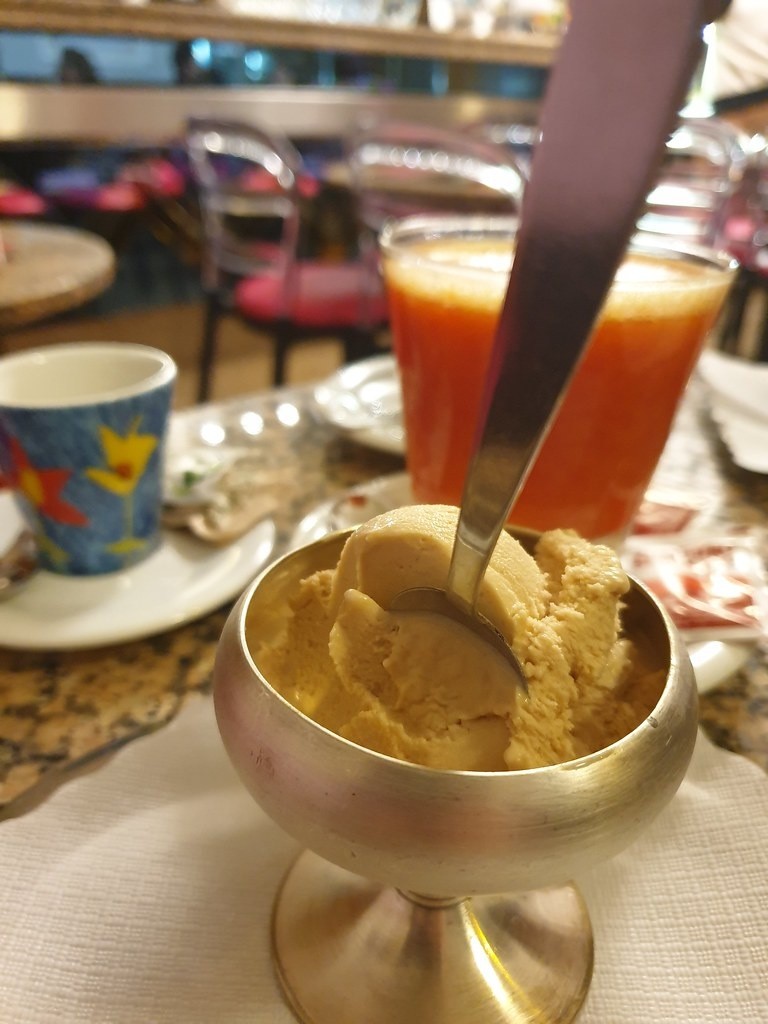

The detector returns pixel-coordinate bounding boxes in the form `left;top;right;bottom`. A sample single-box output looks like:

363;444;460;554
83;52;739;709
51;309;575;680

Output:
1;686;768;1023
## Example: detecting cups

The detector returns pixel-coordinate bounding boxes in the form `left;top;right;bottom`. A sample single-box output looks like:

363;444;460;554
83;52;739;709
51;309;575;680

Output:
1;342;177;576
377;216;737;559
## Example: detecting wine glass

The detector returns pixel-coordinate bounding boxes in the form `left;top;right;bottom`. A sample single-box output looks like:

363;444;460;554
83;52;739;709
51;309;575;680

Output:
211;514;699;1023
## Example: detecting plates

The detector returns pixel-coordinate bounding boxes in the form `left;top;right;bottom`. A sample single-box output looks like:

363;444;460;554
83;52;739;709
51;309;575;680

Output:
288;466;759;692
312;353;402;458
1;487;276;650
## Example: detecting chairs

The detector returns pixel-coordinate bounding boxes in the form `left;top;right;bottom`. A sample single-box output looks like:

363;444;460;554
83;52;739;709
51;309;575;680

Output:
344;102;767;364
185;117;388;402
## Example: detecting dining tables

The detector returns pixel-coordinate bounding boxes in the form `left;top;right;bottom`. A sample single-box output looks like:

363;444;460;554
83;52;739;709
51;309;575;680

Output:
1;350;768;1024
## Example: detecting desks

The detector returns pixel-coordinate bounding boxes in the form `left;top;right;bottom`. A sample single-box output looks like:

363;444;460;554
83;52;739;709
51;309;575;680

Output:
0;213;117;328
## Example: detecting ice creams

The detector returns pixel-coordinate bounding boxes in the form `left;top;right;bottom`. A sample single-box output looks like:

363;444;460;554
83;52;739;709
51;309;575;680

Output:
265;504;668;771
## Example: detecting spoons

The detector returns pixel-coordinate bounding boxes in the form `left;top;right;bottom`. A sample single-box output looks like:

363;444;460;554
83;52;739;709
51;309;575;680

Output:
386;2;715;690
